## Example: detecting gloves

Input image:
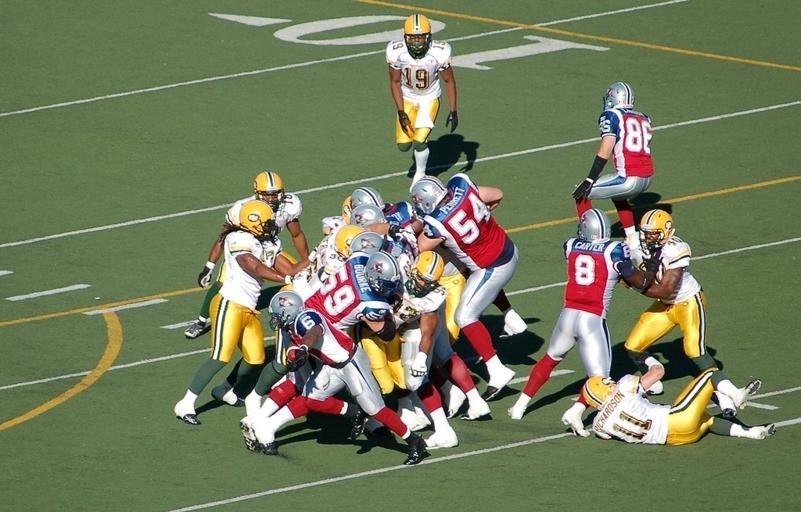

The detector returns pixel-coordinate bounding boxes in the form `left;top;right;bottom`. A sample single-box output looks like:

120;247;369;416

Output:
442;111;462;134
642;243;666;274
407;352;427;391
281;344;309;373
568;175;594;203
397;113;416;142
194;259;216;289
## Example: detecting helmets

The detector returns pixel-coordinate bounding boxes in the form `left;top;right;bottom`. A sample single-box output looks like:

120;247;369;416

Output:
576;208;612;245
582;375;614;409
638;207;675;249
401;14;432;59
602;81;635;113
264;288;307;331
239;170;284;242
336;175;448;297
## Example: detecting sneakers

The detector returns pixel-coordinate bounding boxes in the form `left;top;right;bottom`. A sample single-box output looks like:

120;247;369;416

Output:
211;387;245;407
240;396;278;456
556;402;594;440
403;434;432;465
460;398;491;420
481;368;516;401
444;386;466;419
388;406;431;434
721;380;779;443
173;400;201;425
350;404;369;440
424;427;458;450
638;379;667;396
507;404;526;420
501;309;528;339
184;315;209;340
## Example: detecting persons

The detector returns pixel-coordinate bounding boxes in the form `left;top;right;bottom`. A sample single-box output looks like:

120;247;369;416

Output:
581;351;775;447
623;207;737;418
385;13;458;194
506;207;663;435
569;80;654;236
